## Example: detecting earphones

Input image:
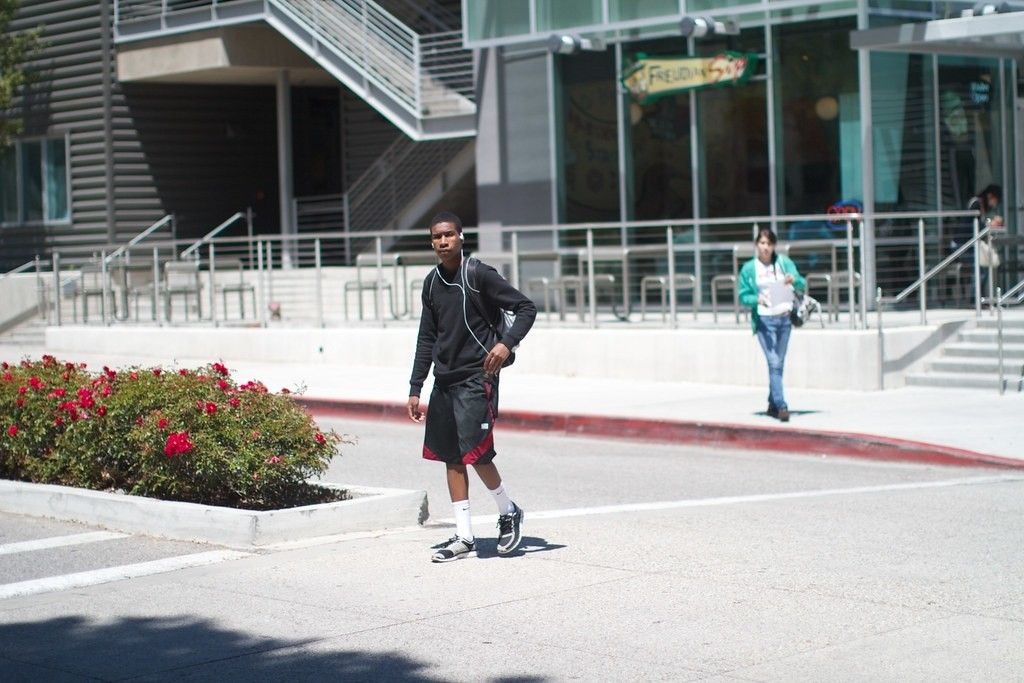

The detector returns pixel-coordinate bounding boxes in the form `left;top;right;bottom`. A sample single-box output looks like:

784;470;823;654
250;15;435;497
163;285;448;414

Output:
432;243;435;248
459;232;465;244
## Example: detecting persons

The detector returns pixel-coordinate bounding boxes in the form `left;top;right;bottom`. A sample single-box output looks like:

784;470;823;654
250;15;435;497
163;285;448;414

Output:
789;210;836;271
407;210;537;563
251;190;269;233
311;146;329;194
737;229;807;422
672;225;707;243
951;184;1002;309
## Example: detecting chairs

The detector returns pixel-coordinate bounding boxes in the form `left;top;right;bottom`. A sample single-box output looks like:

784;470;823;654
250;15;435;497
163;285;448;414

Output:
951;234;986;306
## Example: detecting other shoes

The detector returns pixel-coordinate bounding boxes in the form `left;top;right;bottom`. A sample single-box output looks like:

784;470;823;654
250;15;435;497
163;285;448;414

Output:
767;407;778;417
778;408;789;421
968;302;989;310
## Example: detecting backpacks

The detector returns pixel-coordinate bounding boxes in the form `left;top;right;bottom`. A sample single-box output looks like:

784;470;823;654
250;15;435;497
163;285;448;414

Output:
427;258;520;368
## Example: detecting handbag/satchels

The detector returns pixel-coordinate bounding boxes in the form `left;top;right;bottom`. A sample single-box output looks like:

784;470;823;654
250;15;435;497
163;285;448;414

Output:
790;290;817;327
979;240;999;268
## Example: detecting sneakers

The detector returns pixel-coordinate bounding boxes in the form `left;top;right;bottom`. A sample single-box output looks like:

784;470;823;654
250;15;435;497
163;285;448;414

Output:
495;501;523;554
431;534;479;562
